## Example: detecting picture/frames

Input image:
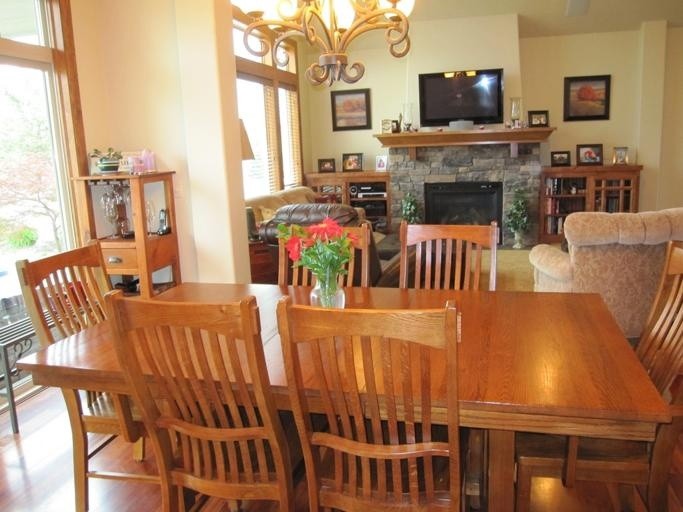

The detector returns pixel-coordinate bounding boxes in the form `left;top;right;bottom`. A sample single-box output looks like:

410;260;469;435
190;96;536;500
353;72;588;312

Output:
563;74;611;122
317;158;335;173
551;151;571;167
376;156;388;171
576;143;603;165
330;87;370;133
528;110;549;126
342;153;362;172
612;146;629;166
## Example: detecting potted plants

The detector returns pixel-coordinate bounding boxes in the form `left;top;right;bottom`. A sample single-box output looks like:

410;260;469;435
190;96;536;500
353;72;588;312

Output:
89;148;122;173
506;187;529;251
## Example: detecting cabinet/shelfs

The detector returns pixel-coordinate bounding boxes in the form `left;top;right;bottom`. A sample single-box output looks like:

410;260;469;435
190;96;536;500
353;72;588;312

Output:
71;167;182;299
306;169;390;217
540;165;644;243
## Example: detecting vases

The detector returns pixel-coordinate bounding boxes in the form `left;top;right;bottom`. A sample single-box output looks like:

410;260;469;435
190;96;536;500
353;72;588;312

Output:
310;271;345;308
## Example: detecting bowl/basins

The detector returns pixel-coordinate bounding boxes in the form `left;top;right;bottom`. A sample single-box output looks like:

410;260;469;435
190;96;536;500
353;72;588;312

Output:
113;282;137;293
448;121;474;130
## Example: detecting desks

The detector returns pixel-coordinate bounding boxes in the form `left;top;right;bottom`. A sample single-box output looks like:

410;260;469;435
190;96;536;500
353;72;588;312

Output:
14;285;672;512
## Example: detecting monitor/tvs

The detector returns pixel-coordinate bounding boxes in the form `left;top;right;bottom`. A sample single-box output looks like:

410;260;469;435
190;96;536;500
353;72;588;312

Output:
419;69;503;126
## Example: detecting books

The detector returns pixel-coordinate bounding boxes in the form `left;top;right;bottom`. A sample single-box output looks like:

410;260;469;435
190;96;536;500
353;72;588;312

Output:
545;177;619;236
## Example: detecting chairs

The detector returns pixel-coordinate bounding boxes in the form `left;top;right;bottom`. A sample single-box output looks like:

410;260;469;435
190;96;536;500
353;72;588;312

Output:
276;223;370;288
15;239;184;512
102;287;306;512
528;207;683;340
399;218;501;292
509;240;683;512
258;200;425;287
274;296;483;512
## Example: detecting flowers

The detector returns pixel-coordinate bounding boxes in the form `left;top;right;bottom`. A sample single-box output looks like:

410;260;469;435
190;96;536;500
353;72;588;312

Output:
275;217;360;308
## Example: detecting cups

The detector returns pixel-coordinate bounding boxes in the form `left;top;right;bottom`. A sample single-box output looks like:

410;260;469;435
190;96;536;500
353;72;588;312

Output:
127;148;157;176
64;281;87;307
503;119;522;129
47;291;67;315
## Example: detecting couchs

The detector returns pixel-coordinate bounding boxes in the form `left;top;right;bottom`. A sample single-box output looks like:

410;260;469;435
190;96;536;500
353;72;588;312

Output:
245;186;388;257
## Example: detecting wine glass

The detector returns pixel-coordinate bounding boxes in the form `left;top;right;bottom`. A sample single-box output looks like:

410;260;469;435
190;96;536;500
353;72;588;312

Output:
98;185;154;239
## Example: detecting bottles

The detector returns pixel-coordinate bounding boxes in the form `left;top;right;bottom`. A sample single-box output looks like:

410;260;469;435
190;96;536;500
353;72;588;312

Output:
509;96;522;127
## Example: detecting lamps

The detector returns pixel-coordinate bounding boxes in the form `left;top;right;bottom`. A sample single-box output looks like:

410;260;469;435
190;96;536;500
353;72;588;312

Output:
230;0;415;88
